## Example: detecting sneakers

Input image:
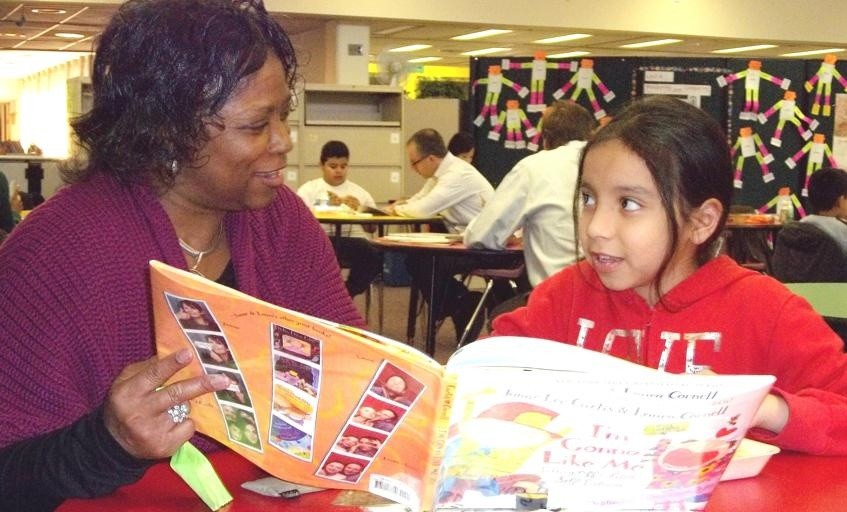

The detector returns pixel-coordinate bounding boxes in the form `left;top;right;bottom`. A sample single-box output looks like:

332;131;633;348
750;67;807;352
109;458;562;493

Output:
451;291;487;344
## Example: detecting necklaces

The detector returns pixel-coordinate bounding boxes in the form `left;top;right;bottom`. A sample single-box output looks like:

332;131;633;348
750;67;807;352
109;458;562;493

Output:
174;217;227;280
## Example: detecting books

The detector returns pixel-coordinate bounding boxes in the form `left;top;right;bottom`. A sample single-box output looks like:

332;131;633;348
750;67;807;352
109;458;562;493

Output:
353;205;392;217
143;255;776;512
384;232;465;244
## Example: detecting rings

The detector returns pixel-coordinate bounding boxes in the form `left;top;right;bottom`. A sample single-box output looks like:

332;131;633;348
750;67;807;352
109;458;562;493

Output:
166;404;187;424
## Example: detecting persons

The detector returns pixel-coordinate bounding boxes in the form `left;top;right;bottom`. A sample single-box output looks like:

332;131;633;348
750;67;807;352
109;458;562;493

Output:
0;170;23;235
411;131;482;233
459;100;603;335
370;376;412;407
295;140;388;301
353;408;398;433
214;371;252;408
318;461;362;483
198;335;238;369
221;405;261;450
490;89;847;461
335;436;381;457
1;0;372;512
771;163;847;347
175;300;220;332
386;125;501;348
